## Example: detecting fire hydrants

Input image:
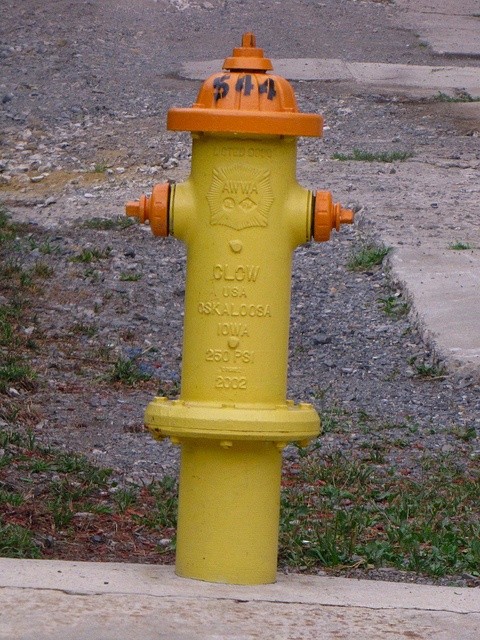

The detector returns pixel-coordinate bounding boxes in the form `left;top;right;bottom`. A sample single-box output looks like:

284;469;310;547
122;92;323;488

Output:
127;32;353;590
